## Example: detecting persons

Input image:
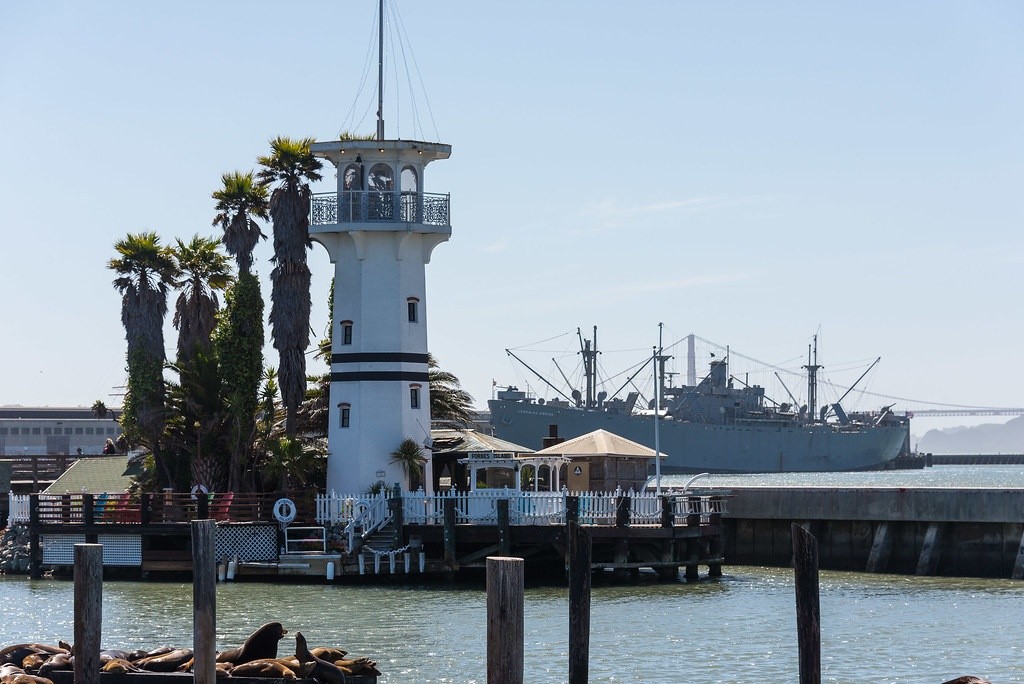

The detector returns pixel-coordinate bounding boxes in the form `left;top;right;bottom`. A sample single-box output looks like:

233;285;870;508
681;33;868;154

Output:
77;448;81;455
103;438;115;454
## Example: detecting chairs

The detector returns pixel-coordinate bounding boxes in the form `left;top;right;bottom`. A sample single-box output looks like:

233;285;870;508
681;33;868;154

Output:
191;491;215;520
209;491;234;521
83;491;156;524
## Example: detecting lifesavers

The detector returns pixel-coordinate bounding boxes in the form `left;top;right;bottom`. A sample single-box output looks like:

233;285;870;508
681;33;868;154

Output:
273;498;296;523
190;485;208;499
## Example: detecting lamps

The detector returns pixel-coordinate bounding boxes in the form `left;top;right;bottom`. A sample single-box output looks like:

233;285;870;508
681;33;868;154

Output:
416;419;433;449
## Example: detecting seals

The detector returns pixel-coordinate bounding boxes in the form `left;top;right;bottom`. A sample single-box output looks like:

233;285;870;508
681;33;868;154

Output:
0;621;382;684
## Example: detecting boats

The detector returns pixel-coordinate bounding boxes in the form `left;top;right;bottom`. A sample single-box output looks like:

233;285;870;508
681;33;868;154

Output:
485;320;912;475
